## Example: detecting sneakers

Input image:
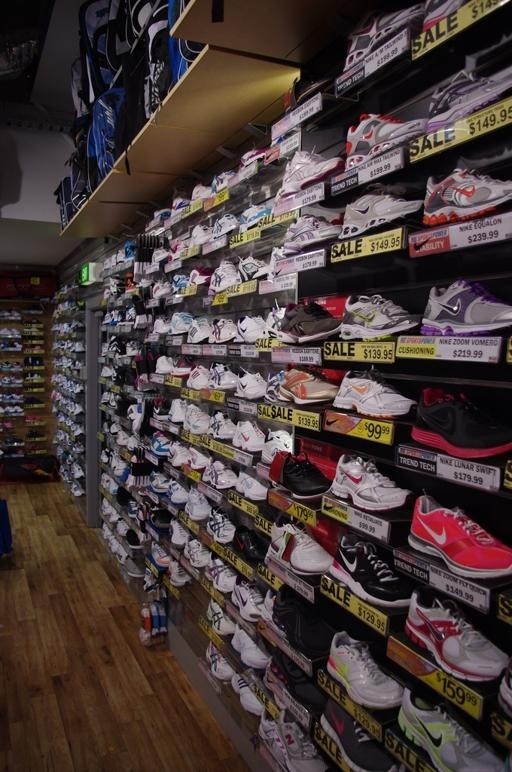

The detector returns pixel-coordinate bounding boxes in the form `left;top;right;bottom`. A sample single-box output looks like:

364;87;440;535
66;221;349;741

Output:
187;267;214;289
232;576;265;622
185;487;212;521
190;184;215;205
269;247;286;280
284;75;332;114
239;150;267;173
184;535;212;568
204;552;237;593
265;649;327;716
202;456;238;489
207;410;236;439
186;364;211;390
234;525;268;564
234;366;267;401
421;281;510;337
279;145;346;199
345;114;429;172
424;167;510;226
264;371;283;404
212;214;239;239
186;317;210;344
205;641;236;681
398;686;507;770
209;318;237;344
262;429;293;465
262;590;288;638
320;698;397;771
278;368;340;405
327;631;403;708
408;495;510;578
209;261;242;293
210;170;239;198
207;362;238;391
338;195;424;240
265;298;286;338
232;421;265;452
235;467;270;501
206;507;236;545
239;204;272;234
340;295;423;340
231;674;264;716
344;4;425;73
406;587;509;682
272;584;332;657
190;224;212;247
268;515;335;575
233;316;265;344
278;303;343;343
232;623;271;668
332;370;417;419
259;709;328;771
329;534;412;607
427;68;510;135
423;2;465;32
0;197;190;590
412;387;510;459
237;255;270;282
330;454;411;510
283;215;343;257
273;451;330;499
187;444;213;470
206;598;235;636
183;404;212;435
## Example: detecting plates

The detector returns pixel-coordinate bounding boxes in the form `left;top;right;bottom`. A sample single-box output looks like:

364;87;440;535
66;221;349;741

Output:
96;259;157;592
0;297;53;482
49;284;101;527
269;0;512;772
146;141;302;720
